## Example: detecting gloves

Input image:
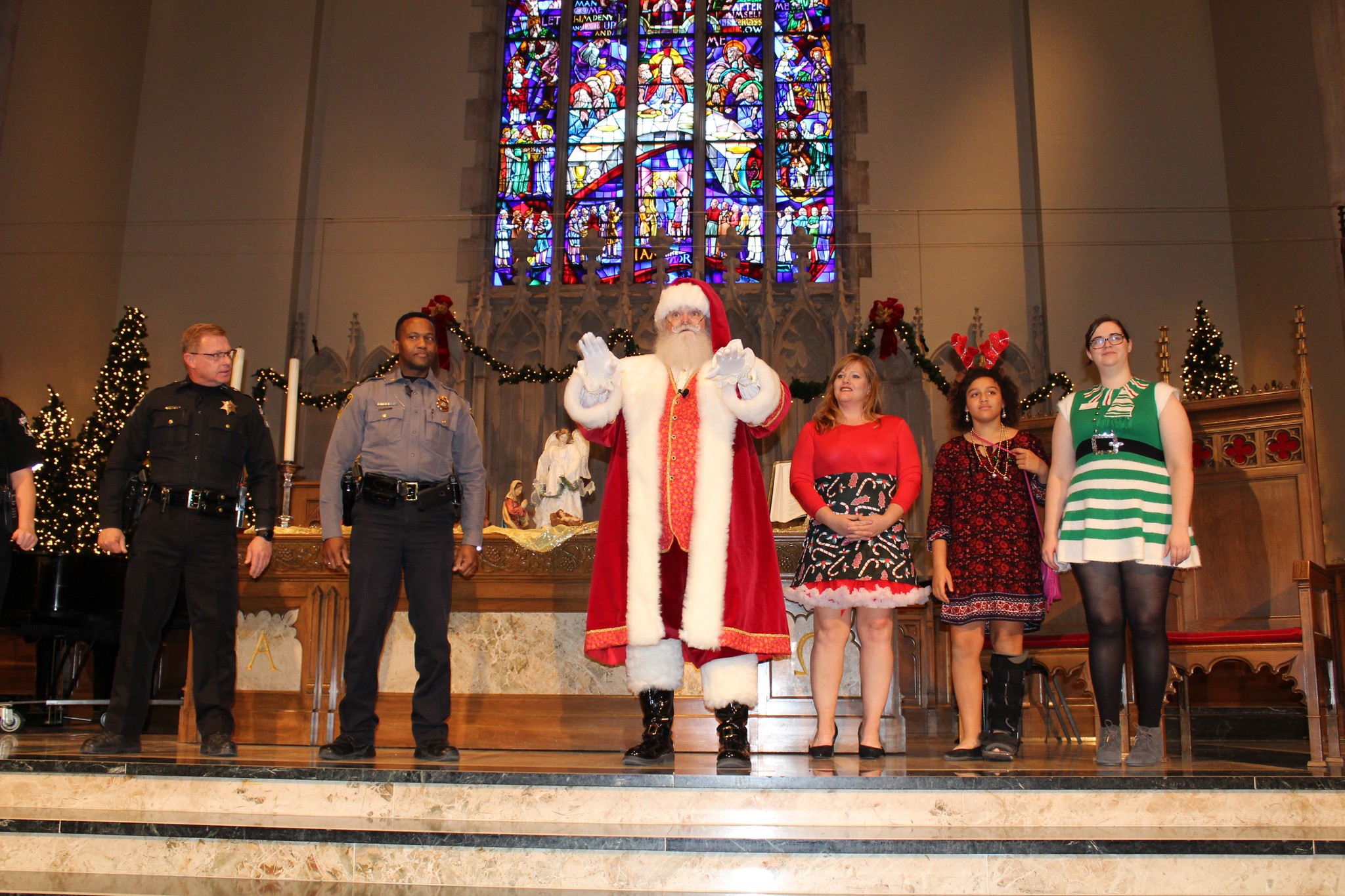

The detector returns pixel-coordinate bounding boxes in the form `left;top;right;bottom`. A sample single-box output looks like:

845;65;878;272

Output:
578;331;617;383
705;346;746;378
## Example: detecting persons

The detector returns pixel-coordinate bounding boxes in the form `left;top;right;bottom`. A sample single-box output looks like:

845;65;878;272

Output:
502;480;529;529
555;509;580;523
782;354;934;762
561;277;792;771
1040;312;1202;769
317;311;489;761
0;393;40;630
925;367;1063;763
81;324;281;756
534;427;592;528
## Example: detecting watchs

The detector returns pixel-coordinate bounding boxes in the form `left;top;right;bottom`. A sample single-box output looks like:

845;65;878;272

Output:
255;528;275;541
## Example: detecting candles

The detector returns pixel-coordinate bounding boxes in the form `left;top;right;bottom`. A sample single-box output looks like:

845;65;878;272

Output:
229;344;248;392
283;353;301;463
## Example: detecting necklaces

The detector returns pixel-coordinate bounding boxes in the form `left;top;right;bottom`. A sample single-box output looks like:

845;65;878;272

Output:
972;425;1012;481
969;423;1003;478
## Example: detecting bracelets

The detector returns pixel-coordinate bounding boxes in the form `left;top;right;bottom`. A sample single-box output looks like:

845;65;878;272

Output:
471;545;482;553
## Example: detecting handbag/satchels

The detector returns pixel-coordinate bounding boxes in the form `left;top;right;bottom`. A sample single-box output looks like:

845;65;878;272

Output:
1040;557;1062;612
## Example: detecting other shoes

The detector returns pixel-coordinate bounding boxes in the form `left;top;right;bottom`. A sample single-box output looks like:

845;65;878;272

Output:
944;743;984;761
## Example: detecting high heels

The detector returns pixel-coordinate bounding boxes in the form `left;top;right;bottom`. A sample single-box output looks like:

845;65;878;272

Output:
858;722;886;760
808;721;838;758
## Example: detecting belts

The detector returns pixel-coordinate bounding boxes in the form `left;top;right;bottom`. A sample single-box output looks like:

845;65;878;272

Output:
398;482;444;502
150;487;203;510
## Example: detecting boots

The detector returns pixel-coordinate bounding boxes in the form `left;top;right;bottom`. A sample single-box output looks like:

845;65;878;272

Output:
714;701;752;769
1095;725;1123;766
622;687;675;766
1124;726;1164;767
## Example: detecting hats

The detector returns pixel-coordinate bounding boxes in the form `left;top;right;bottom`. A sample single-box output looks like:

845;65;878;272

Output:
654;277;731;355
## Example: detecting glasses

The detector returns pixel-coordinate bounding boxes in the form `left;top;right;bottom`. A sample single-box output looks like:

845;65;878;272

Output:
190;349;237;362
665;313;706;328
1089;333;1125;349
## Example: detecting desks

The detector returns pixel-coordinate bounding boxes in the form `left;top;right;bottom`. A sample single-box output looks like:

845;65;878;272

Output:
166;511;969;755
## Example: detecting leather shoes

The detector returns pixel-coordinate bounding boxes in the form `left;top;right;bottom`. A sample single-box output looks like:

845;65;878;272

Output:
413;739;460;762
199;732;238;757
317;734;376;760
80;730;141;754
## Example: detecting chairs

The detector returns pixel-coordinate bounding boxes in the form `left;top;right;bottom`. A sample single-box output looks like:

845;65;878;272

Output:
951;296;1345;778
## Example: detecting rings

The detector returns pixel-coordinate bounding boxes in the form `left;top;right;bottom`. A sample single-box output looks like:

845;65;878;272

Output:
326;562;332;566
